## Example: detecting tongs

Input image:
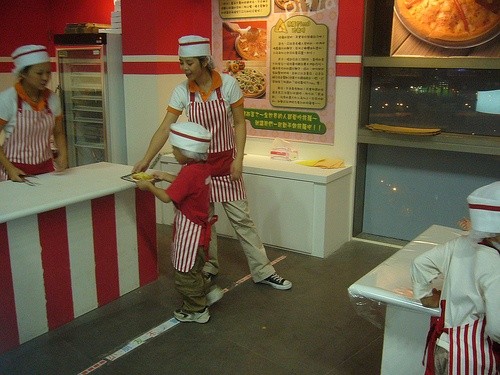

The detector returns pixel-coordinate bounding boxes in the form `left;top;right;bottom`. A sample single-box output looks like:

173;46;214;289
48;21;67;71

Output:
17;174;38;186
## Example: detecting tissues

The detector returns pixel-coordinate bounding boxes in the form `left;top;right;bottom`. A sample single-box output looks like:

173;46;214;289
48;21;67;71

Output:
269;139;299;161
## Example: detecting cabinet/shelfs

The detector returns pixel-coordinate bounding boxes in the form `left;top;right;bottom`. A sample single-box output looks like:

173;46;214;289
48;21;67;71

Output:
61;59;105;153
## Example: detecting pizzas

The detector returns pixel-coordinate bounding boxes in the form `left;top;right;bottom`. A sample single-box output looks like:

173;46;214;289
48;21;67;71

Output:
235;27;267;60
394;0;500;47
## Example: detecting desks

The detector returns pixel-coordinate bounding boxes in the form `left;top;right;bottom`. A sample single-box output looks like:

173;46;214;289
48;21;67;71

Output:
159;146;354;260
0;161;176;353
347;224;500;375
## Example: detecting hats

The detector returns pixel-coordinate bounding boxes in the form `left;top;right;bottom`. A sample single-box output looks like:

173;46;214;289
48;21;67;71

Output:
177;35;211;59
468;180;500;233
11;44;49;74
170;122;211;152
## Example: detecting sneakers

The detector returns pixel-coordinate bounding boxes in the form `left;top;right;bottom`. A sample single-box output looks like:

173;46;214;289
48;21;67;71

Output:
261;273;293;291
202;271;212;283
173;307;210;324
206;287;223;305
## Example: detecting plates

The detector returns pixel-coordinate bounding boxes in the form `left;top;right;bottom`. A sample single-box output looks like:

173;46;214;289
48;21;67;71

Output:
393;6;500;50
254;90;266;99
120;172;160;184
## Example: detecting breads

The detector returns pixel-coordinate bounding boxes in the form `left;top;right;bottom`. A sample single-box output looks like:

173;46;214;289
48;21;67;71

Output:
131;172;153;180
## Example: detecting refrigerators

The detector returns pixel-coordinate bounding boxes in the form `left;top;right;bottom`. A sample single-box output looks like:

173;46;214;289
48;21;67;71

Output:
53;32;127;168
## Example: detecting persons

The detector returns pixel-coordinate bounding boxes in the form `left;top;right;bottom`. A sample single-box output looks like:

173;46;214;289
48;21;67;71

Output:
132;35;292;289
0;45;68;182
410;181;500;375
136;122;218;323
